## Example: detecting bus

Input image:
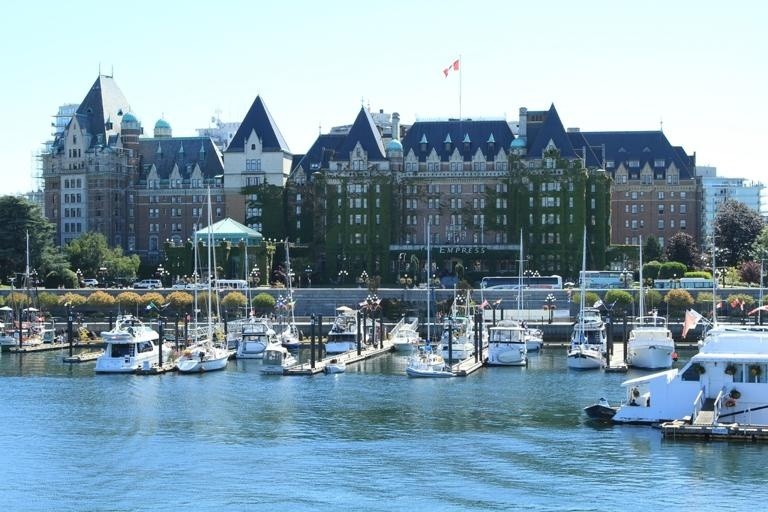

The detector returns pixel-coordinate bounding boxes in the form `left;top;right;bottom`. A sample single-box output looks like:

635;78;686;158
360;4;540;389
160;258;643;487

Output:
214;279;248;290
482;274;564;290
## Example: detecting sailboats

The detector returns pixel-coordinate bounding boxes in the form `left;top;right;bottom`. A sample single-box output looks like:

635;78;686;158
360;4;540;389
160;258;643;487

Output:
173;183;238;373
0;229;102;350
193;223;300;375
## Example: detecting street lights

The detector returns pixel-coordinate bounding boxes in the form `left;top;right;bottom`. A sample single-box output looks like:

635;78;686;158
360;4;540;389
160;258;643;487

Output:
544;293;557;324
338;270;348;280
360;270;369;288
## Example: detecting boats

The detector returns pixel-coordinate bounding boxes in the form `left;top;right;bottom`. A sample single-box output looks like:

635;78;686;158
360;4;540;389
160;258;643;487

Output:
325;310;387;352
62;347;105;363
391;222;543;377
92;302;172;373
567;223;768;422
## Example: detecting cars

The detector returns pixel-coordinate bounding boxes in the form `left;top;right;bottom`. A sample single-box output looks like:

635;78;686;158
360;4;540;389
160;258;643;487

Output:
172;282;192;289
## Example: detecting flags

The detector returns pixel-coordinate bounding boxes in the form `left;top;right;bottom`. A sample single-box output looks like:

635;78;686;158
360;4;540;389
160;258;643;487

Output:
689;309;702;330
682;309;696;338
441;59;460;78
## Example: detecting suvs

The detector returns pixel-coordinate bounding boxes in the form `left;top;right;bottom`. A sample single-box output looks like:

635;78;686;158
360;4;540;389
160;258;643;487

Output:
133;279;162;289
81;279;99;288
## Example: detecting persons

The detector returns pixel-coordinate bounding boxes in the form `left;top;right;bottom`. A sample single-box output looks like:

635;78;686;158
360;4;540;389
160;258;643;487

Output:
633;390;647;407
